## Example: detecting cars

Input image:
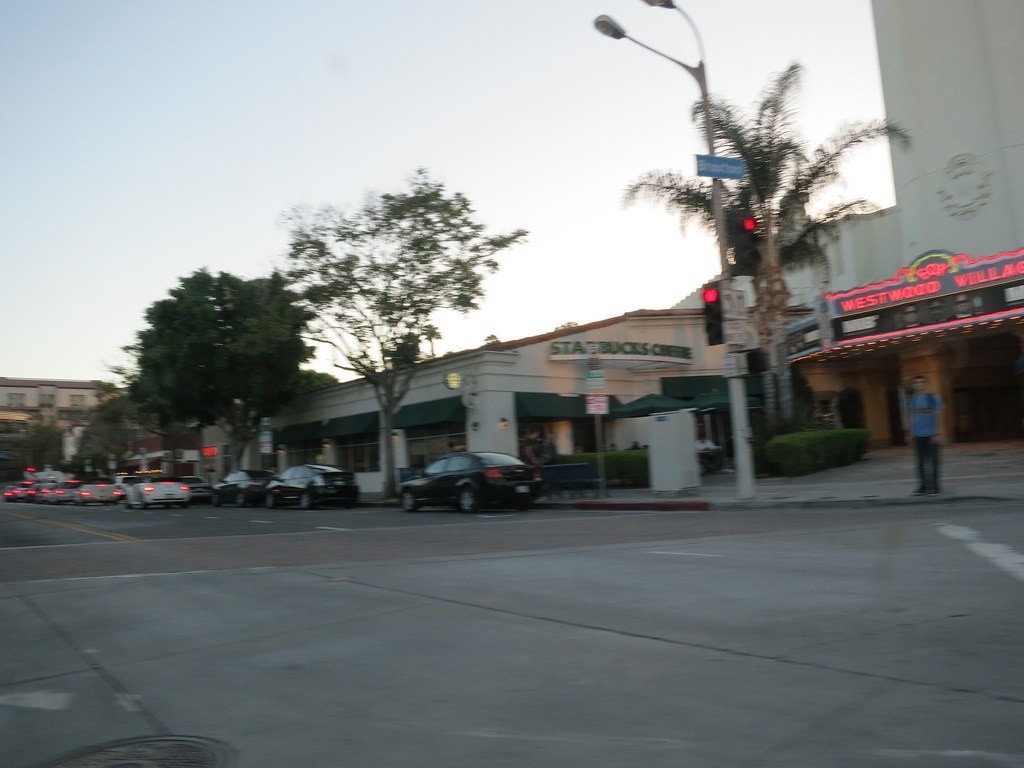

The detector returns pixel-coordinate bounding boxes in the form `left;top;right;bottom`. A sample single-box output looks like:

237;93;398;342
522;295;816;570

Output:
209;470;274;507
263;464;357;510
397;451;543;516
3;475;211;511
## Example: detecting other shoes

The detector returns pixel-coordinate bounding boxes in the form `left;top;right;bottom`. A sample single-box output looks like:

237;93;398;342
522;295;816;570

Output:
928;488;940;497
911;487;927;495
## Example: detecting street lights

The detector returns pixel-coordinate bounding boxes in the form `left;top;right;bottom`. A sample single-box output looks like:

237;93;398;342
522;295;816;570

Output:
594;1;761;500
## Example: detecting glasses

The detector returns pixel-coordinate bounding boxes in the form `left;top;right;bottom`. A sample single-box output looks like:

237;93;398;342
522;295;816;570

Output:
912;380;924;384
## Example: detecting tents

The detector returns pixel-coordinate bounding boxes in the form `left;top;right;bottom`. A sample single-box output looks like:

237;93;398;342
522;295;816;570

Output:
610;388;760;418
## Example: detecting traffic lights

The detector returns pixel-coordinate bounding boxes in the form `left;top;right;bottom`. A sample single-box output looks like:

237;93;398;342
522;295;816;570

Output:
699;279;724;343
726;205;762;277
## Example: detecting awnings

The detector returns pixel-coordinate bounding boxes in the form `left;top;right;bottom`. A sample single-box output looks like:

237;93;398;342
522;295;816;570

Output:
274;393;461;446
515;392;623;421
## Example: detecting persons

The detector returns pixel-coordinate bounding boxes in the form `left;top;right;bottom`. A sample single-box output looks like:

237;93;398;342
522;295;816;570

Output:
905;377;947;495
901;292;982;328
521;429;732;475
